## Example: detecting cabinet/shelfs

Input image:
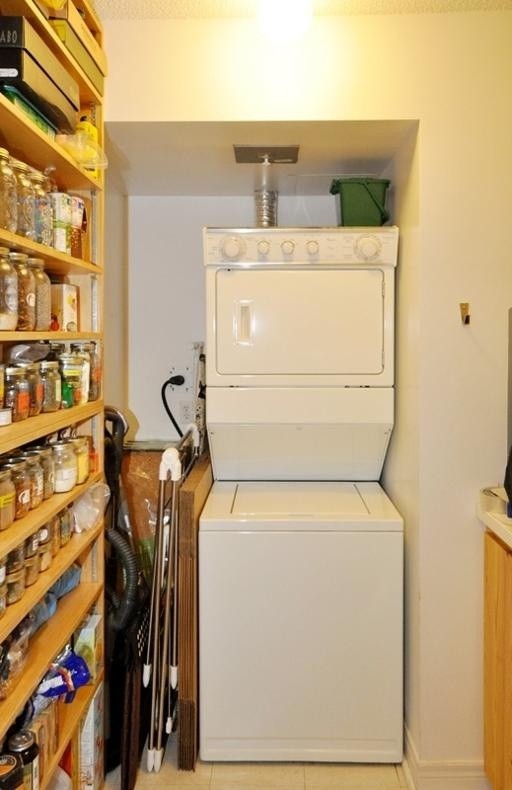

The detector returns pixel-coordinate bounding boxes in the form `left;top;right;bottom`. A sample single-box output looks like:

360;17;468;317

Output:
1;0;108;789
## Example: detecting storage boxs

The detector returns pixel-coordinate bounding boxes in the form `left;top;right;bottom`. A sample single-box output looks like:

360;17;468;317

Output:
0;0;107;136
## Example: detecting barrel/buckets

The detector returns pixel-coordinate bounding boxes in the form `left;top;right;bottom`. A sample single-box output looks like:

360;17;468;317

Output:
328;176;391;227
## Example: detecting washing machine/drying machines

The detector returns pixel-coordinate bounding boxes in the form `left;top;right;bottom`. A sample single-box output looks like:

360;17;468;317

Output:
196;483;407;768
202;226;399;482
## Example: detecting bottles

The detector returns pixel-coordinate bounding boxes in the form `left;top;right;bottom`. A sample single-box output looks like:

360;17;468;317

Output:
0;145;55;248
0;246;52;332
47;342;102;402
0;501;76;621
0;435;90;532
3;360;62;422
